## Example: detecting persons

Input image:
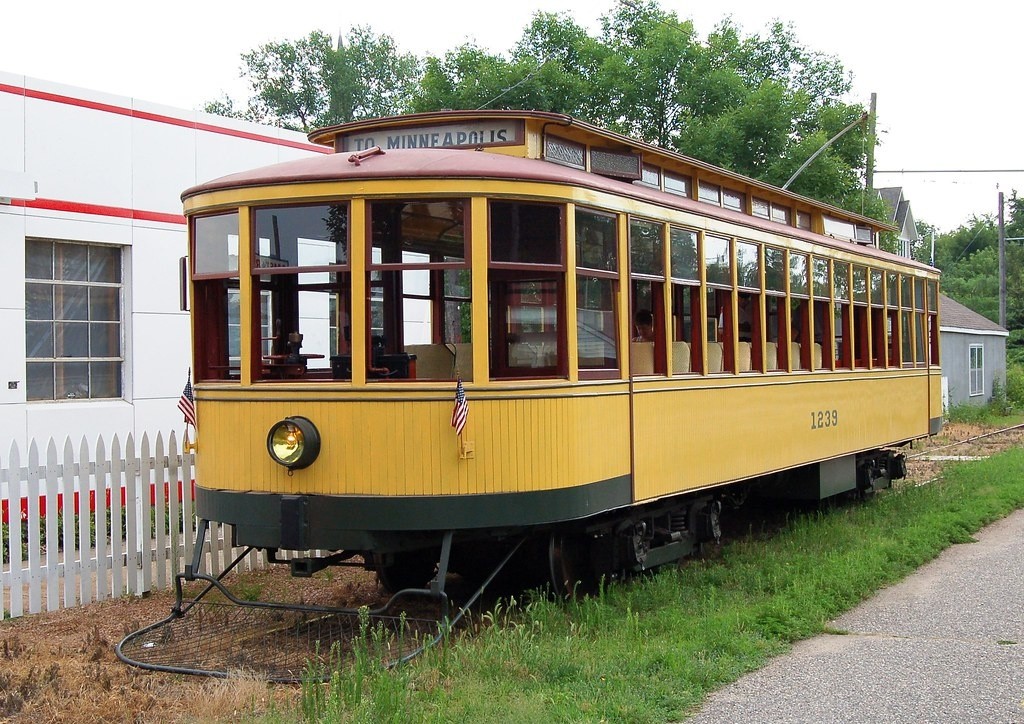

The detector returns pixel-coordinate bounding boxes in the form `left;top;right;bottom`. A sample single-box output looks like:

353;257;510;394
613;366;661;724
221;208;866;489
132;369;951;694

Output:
632;310;654;342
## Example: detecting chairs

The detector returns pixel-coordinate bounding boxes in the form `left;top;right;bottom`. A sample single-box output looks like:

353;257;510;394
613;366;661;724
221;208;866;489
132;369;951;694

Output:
630;341;822;374
404;339;605;379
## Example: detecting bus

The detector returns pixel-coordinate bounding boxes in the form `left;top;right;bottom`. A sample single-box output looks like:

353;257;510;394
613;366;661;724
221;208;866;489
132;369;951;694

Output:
113;108;947;612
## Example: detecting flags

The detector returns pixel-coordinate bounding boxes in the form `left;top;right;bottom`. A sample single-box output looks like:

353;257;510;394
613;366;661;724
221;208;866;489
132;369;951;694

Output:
450;378;469;437
177;381;196;431
